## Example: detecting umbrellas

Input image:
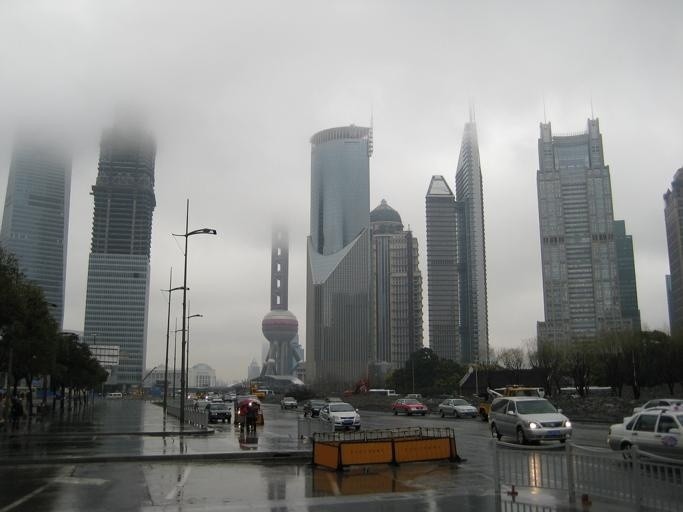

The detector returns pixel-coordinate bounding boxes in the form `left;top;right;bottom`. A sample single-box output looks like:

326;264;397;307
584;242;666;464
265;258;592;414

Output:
239;399;260;407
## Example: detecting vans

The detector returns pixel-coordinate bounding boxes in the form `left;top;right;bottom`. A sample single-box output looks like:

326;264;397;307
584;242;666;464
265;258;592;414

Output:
106;393;122;400
489;396;572;445
405;394;422;399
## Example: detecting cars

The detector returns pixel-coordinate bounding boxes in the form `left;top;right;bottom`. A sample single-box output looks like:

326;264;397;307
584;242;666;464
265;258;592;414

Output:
281;396;297;409
188;392;264;425
304;397;362;431
438;398;479;418
607;399;683;460
391;398;428;416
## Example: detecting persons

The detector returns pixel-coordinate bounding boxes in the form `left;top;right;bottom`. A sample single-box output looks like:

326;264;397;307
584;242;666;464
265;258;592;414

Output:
10;398;24;430
1;394;8;431
240;403;258;432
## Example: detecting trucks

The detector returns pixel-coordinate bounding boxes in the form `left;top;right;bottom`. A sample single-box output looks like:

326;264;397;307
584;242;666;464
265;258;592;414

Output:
479;386;540;420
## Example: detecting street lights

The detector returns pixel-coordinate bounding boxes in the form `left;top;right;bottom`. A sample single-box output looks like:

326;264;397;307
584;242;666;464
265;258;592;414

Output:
161;198;217;427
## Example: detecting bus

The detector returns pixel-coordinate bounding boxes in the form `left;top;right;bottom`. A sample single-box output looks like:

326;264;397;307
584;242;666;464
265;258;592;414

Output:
9;386;37;400
368;389;395;396
